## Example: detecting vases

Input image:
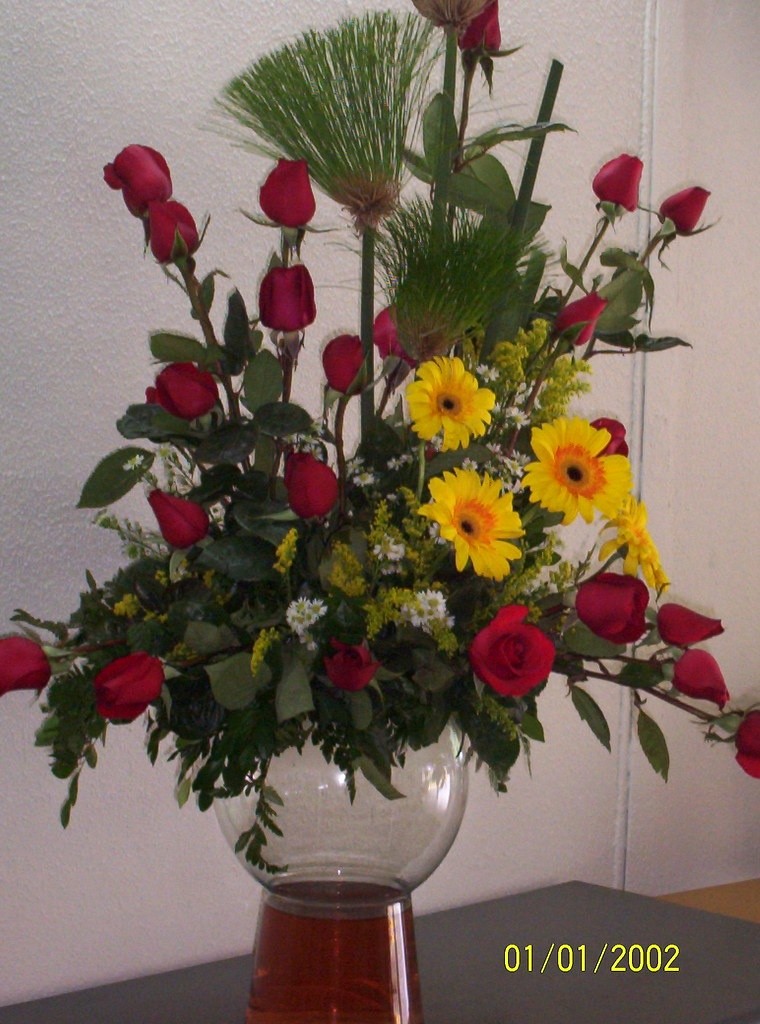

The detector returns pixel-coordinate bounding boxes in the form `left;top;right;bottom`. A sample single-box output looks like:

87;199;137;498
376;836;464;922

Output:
211;715;471;1023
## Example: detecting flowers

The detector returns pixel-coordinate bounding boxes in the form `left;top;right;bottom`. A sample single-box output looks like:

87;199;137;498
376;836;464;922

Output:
1;0;760;872
